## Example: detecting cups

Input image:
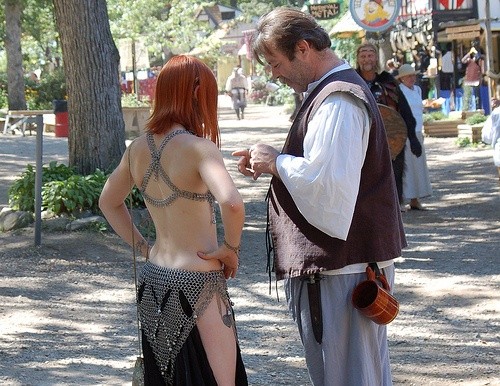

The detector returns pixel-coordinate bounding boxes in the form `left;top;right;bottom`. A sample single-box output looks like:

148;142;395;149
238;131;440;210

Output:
351;266;399;325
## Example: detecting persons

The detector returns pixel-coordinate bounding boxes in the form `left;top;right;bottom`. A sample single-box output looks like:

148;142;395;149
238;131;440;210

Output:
99;55;249;386
225;67;249;120
393;64;433;213
231;5;408;386
385;37;491;116
355;44;423;201
481;98;500;181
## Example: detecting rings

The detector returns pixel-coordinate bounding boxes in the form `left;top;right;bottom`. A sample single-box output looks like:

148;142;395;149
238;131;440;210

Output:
247;147;250;151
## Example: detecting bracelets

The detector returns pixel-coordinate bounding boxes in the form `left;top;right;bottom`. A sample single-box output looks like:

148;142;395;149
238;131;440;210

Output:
134;238;146;253
223;237;240;251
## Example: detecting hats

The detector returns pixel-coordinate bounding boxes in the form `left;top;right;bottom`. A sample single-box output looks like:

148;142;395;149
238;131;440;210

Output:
394;63;421;79
